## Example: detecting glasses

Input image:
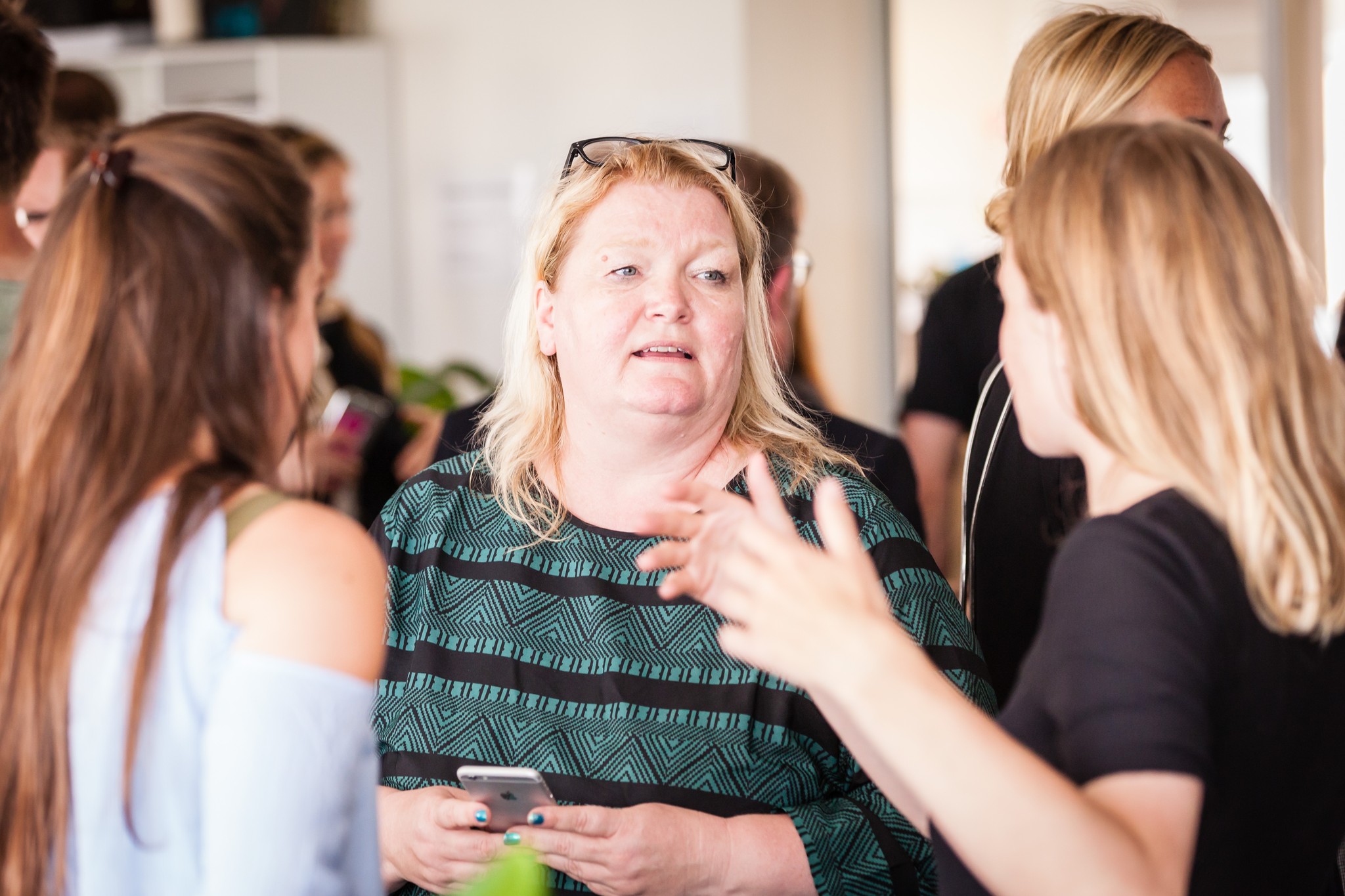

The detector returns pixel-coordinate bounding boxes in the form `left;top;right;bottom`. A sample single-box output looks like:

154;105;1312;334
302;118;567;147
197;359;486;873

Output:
789;252;814;287
563;136;739;182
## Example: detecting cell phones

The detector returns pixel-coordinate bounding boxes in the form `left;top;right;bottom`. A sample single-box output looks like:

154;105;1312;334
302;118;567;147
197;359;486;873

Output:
456;765;559;833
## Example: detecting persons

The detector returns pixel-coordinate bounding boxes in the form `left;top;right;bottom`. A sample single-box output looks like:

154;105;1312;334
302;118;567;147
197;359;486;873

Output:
0;0;1345;896
637;120;1345;896
372;136;998;896
